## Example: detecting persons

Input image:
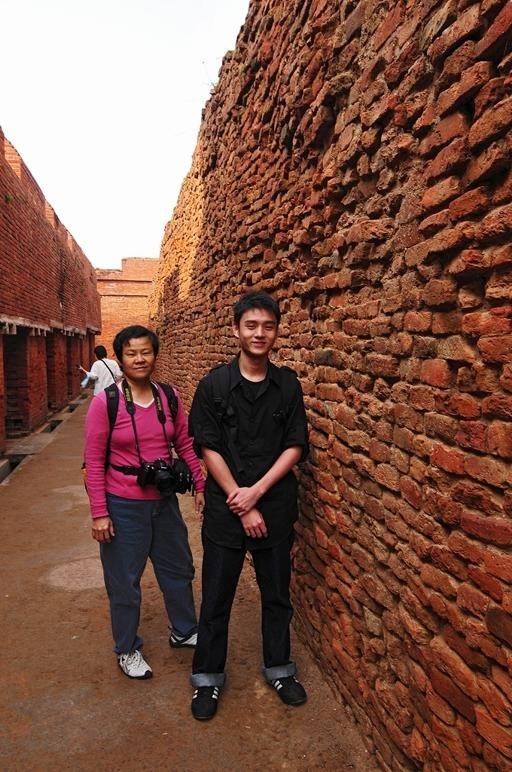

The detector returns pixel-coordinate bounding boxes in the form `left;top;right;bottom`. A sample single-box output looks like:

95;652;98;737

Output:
83;326;206;680
78;345;124;395
186;294;310;727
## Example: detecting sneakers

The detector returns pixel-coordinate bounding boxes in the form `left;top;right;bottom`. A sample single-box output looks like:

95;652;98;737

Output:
267;675;307;705
191;684;220;720
169;630;198;649
117;649;153;680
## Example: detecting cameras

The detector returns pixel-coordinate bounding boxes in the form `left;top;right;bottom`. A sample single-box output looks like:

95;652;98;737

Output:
137;458;178;496
